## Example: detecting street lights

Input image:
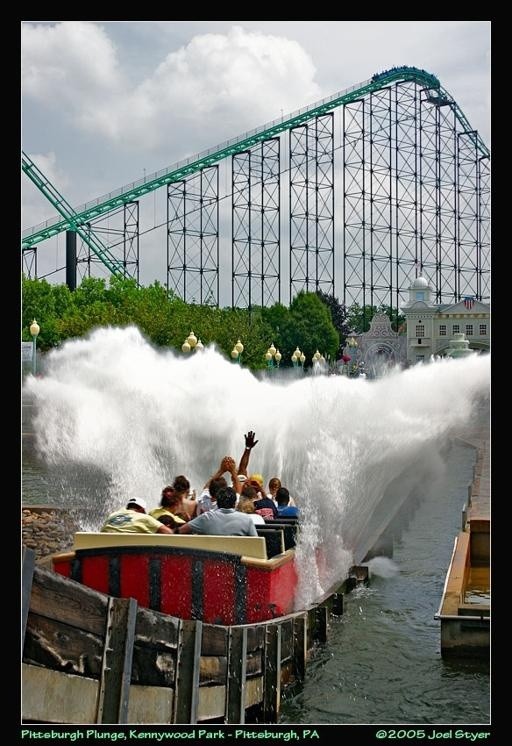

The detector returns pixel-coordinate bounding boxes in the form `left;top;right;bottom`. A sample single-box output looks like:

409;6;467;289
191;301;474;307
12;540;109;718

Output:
291;346;306;377
29;317;40;378
181;329;204;358
311;349;325;375
264;341;283;379
348;337;358;353
230;338;244;365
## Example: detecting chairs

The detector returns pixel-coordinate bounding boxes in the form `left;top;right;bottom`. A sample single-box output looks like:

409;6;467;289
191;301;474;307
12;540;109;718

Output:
165;514;298;560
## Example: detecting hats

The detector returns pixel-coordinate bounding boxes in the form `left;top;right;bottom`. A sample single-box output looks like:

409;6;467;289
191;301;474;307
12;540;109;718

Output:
126;497;148;514
249;474;264;488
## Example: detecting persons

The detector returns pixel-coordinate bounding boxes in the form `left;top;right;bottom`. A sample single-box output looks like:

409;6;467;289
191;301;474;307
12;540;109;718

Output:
99;497;174;534
150;431;301;537
